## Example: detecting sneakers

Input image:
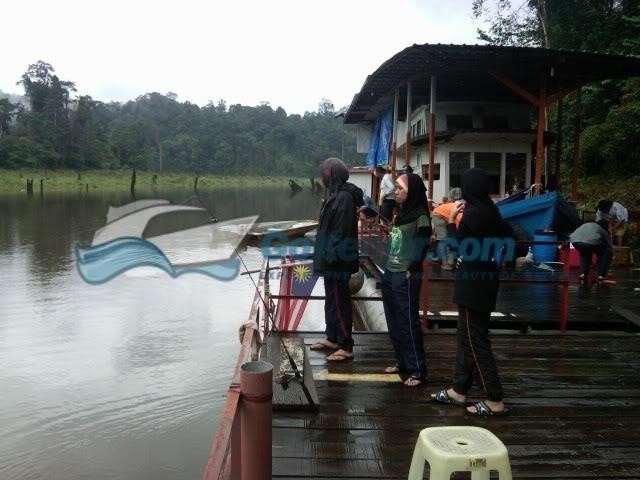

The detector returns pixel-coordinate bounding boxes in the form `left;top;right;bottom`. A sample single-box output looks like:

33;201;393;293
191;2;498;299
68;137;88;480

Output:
441;264;453;270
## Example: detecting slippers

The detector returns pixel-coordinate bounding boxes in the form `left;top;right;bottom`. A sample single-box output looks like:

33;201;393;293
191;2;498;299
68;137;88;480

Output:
385;363;401;374
430;388;467;405
406;376;424;387
464;401;509;416
310;342;338;350
327;353;354;363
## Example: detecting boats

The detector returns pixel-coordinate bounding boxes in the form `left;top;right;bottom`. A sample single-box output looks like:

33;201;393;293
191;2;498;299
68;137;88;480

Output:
495;183;579;240
218;220;319;240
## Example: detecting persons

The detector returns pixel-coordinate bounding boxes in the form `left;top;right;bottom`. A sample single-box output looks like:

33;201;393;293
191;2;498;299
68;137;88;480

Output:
596;200;629;246
381;173;433;387
310;158;366;363
431;168;512;417
356;188;378;229
432;203;463;270
570;219;613;280
376;166;395;233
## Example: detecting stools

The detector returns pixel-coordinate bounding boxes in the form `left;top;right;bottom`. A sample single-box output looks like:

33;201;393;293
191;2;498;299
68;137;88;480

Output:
408;425;512;480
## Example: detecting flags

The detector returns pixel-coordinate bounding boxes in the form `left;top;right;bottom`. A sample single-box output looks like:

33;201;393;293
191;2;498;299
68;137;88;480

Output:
273;253;320;331
